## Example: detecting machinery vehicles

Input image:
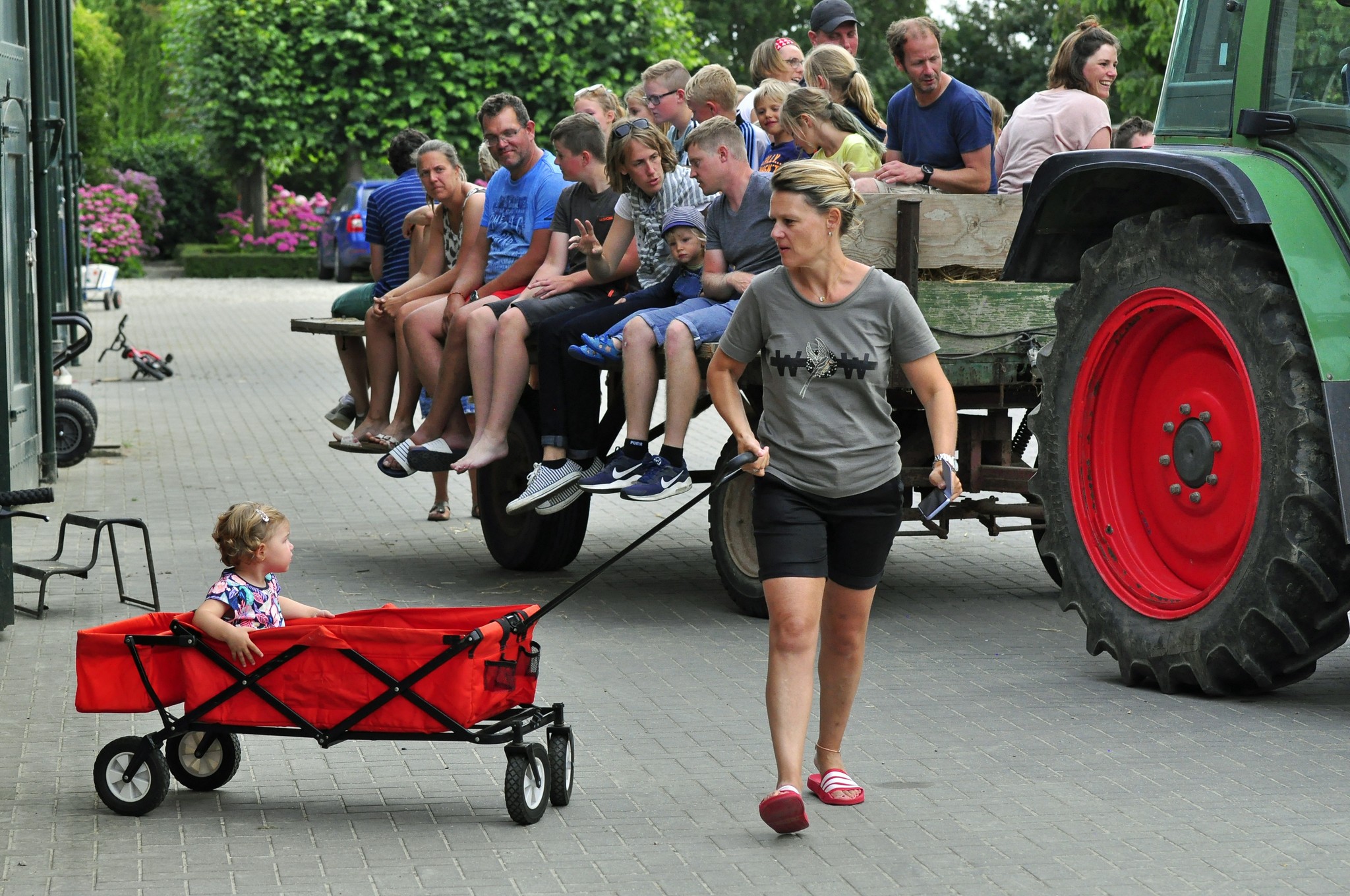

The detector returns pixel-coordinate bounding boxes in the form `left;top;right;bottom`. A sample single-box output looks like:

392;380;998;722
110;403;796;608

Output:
290;0;1350;700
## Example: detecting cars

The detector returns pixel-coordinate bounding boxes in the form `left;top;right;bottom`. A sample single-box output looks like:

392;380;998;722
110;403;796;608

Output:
312;179;400;282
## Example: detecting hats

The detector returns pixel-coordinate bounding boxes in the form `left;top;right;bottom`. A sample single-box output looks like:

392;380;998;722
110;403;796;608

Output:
810;0;862;33
661;222;695;233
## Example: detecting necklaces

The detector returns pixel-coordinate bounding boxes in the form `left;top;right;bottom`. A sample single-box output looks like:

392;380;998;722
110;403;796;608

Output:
808;260;847;302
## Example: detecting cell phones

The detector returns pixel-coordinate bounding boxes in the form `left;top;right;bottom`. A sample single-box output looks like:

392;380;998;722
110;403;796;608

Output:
918;487;948;520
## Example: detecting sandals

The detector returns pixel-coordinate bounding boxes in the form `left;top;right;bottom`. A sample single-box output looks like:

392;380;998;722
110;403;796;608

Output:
325;393;356;430
568;344;604;367
472;501;482;519
428;501;451;520
581;333;622;360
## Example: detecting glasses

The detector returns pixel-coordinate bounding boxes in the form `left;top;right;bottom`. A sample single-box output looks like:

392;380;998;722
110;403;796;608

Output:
611;117;649;139
483;125;522;147
782;58;803;69
642;89;677;105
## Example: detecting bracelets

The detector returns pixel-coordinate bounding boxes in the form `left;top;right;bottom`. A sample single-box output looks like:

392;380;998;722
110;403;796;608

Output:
447;292;466;300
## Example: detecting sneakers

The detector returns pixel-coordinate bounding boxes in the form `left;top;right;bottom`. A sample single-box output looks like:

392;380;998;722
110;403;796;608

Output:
505;460;581;516
535;455;605;515
578;446;652;494
620;455;692;502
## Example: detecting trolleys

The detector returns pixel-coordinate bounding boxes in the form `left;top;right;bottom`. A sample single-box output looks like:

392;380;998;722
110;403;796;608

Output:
78;447;767;828
75;263;124;311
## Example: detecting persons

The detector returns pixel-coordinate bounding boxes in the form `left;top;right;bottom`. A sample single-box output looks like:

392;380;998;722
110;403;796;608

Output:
191;504;336;666
324;0;1156;522
708;157;960;832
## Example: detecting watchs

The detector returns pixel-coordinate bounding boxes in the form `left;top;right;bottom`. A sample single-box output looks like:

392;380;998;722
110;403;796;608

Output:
470;289;480;302
920;164;933;185
929;454;958;474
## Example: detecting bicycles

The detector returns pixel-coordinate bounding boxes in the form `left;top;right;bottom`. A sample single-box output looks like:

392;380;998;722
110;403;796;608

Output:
97;314;174;381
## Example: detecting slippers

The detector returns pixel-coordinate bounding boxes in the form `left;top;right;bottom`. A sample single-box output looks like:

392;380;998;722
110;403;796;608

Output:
807;767;865;806
328;432;387;454
376;438;418;478
758;785;810;834
407;437;472;472
362;433;400;450
